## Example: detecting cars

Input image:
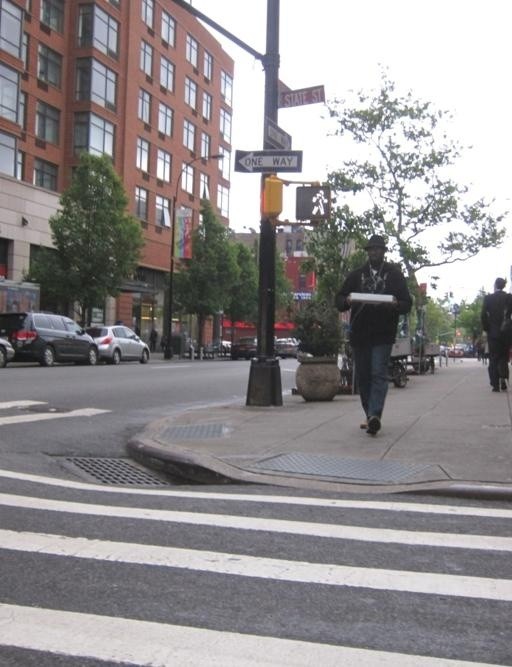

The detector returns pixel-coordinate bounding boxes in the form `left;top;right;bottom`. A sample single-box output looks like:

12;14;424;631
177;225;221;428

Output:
437;343;475;358
220;335;303;360
0;336;17;368
84;324;151;365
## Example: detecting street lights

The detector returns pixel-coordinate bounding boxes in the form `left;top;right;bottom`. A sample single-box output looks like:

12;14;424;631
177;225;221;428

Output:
451;303;461;362
161;152;225;360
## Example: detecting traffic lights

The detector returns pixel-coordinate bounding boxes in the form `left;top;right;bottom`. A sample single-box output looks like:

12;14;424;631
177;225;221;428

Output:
295;185;332;220
258;177;282;218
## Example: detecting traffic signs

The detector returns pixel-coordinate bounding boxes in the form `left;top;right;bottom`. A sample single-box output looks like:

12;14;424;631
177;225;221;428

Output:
278;84;326;110
278;80;294;93
262;116;292;151
234;149;303;174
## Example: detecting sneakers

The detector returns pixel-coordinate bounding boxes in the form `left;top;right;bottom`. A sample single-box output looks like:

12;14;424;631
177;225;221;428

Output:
366;416;380;435
501;385;507;390
492;386;500;392
360;419;368;428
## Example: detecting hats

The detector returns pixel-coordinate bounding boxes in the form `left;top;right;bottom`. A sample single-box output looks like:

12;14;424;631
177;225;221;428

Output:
362;235;388;252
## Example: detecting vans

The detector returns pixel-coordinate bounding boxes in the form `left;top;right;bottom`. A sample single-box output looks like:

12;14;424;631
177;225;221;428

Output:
0;311;101;367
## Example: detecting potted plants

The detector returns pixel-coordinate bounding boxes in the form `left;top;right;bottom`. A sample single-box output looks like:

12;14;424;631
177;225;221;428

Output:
288;295;345;401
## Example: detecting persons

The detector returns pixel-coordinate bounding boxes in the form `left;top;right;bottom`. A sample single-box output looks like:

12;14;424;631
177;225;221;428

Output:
472;276;512;395
147;326;159;354
332;234;414;437
